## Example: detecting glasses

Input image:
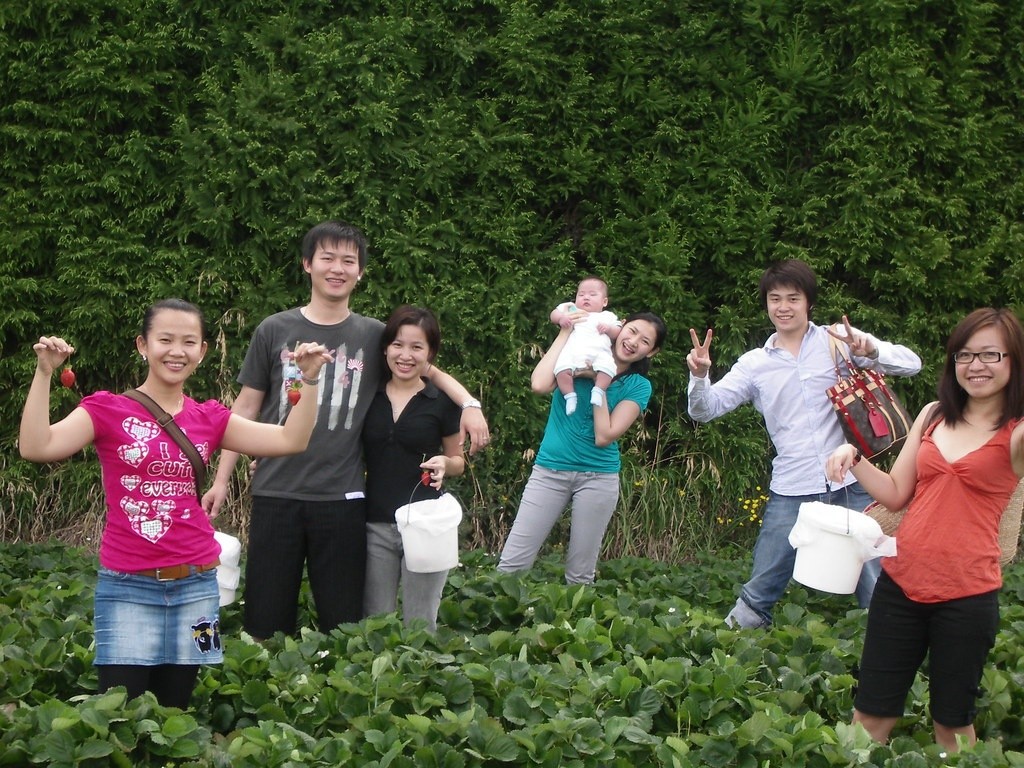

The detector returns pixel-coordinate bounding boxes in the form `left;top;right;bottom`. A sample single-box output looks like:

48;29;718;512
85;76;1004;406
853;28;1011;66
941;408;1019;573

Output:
952;351;1010;363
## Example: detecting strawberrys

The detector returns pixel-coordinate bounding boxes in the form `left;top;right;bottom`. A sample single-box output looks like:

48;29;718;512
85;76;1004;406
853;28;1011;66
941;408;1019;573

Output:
422;472;431;486
60;368;75;388
288;389;301;406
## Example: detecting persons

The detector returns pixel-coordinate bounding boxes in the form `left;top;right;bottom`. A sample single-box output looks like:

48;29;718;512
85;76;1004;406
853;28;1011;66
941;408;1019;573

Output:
202;223;492;647
495;310;665;586
550;277;623;417
826;308;1023;753
686;261;921;634
358;305;466;629
18;299;333;710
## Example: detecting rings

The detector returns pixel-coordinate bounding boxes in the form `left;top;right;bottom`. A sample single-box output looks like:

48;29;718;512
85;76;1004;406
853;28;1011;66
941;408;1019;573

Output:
482;437;487;439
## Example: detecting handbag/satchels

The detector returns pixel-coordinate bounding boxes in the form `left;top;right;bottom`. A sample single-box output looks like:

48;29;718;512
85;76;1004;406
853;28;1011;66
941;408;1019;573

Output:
861;474;1024;576
825;321;914;461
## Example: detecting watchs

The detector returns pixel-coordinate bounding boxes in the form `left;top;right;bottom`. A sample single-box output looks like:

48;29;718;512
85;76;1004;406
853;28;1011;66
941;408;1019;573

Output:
461;400;482;409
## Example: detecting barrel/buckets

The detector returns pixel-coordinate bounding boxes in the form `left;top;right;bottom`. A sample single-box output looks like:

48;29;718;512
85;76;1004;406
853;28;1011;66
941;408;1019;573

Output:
395;474;463;573
792;480;883;594
213;513;242;607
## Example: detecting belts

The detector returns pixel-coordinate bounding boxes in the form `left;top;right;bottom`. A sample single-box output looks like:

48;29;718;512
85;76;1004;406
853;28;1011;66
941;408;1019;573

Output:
132;556;221;582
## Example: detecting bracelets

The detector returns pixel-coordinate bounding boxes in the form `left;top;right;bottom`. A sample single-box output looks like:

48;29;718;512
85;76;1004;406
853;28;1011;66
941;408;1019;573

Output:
300;370;321;384
850;449;863;470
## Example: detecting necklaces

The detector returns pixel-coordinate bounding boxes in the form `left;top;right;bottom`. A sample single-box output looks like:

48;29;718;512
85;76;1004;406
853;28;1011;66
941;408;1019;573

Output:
143;383;183;417
389;377;419;413
303;305;308;318
